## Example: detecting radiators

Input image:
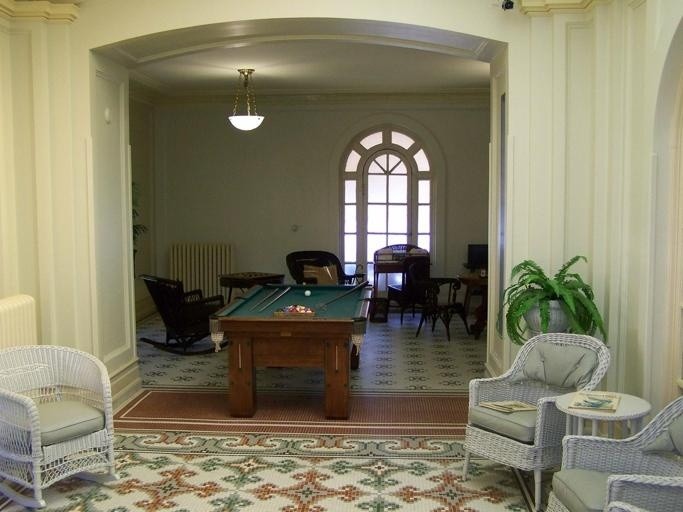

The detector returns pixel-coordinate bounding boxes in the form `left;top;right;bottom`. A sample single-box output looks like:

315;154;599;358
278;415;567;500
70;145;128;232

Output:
169;241;231;304
0;293;40;414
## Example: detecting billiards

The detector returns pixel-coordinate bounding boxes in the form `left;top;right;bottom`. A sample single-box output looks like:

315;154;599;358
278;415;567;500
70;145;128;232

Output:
304;290;310;296
277;304;312;313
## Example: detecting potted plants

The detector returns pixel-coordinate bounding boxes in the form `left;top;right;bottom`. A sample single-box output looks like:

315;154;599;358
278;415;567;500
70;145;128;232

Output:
492;254;610;348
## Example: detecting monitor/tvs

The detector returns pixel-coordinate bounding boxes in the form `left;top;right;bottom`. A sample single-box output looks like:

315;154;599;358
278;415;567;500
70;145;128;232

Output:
468;244;488;279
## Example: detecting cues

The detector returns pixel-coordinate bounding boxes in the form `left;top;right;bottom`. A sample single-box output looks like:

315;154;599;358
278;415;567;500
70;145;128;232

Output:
319;281;369;306
249;288;278;312
259;287;291;312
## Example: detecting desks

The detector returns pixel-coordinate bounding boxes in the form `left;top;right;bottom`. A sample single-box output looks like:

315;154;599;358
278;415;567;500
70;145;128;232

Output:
553;388;653;437
207;281;374;420
368;244;430;324
220;272;286;305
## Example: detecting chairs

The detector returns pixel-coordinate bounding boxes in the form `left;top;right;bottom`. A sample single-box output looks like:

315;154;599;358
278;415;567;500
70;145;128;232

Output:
415;268;489;341
136;272;233;354
460;332;611;510
0;345;118;508
286;250;365;289
383;256;432;324
541;393;682;510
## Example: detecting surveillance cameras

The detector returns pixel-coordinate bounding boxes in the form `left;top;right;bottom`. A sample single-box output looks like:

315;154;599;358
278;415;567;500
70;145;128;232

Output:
502;0;514;11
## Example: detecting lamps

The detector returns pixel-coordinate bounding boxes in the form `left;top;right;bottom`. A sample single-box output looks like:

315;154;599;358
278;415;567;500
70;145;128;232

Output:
227;67;264;131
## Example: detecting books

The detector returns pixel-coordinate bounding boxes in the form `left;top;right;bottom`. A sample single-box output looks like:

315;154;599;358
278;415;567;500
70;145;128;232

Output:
568;392;621;412
479;401;538;412
